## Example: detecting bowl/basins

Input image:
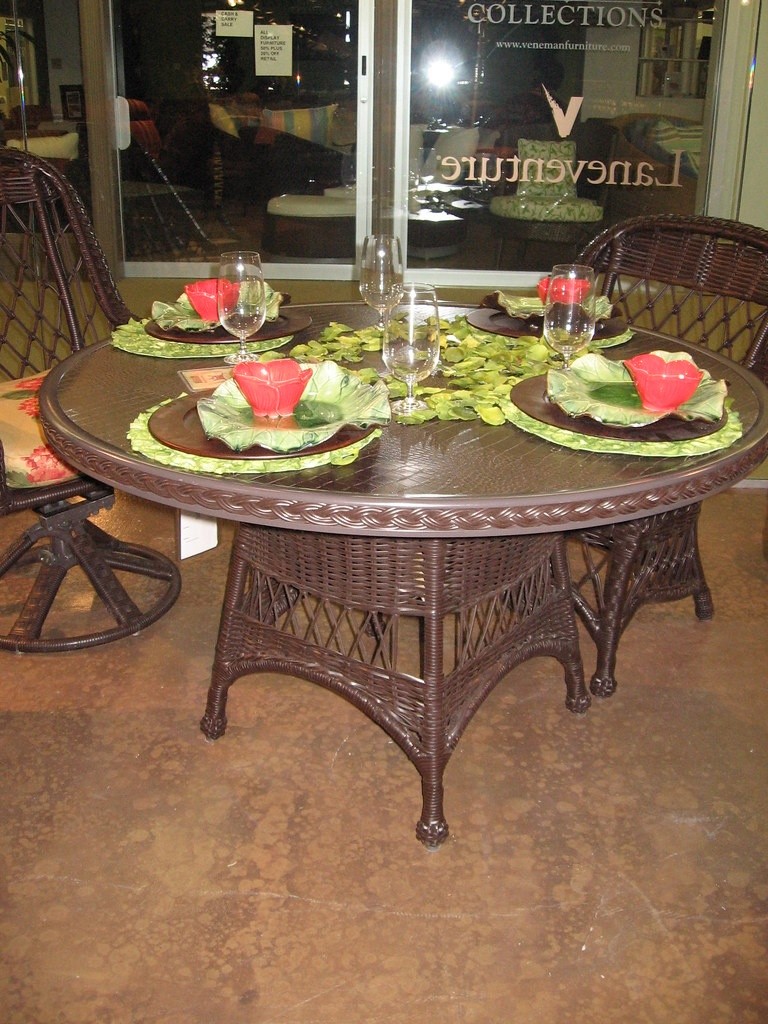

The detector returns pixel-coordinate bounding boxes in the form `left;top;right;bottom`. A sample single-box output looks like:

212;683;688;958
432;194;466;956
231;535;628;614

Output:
231;359;313;420
624;354;704;413
536;277;591;305
183;279;240;322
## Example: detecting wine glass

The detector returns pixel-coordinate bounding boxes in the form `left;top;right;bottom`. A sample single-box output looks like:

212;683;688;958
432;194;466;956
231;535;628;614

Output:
543;264;595;370
359;235;404;331
382;283;440;415
218;250;266;364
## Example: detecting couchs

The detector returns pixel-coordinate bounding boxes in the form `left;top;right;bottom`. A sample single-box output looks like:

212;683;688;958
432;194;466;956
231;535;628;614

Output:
609;111;707;222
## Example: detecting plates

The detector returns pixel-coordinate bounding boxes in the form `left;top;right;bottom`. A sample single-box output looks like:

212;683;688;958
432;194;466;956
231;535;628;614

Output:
147;389;377;460
466;308;629;340
510;374;728;442
145;308;312;343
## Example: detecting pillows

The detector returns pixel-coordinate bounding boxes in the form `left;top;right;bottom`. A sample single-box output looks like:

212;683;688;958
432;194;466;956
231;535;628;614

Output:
6;131;81;160
421;123;481;186
465;126;501;147
260;100;356;168
206;100;243;142
410;123;422;174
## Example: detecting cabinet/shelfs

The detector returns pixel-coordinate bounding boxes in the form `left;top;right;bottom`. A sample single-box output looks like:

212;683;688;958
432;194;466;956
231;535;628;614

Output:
639;17;713;62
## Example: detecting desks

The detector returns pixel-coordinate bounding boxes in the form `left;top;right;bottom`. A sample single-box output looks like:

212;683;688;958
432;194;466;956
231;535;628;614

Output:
42;297;768;850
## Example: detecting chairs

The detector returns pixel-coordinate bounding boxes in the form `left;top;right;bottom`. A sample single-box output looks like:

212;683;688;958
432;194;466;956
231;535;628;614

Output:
554;120;620;206
123;95;169;151
538;209;768;702
488;137;605;273
0;139;184;657
